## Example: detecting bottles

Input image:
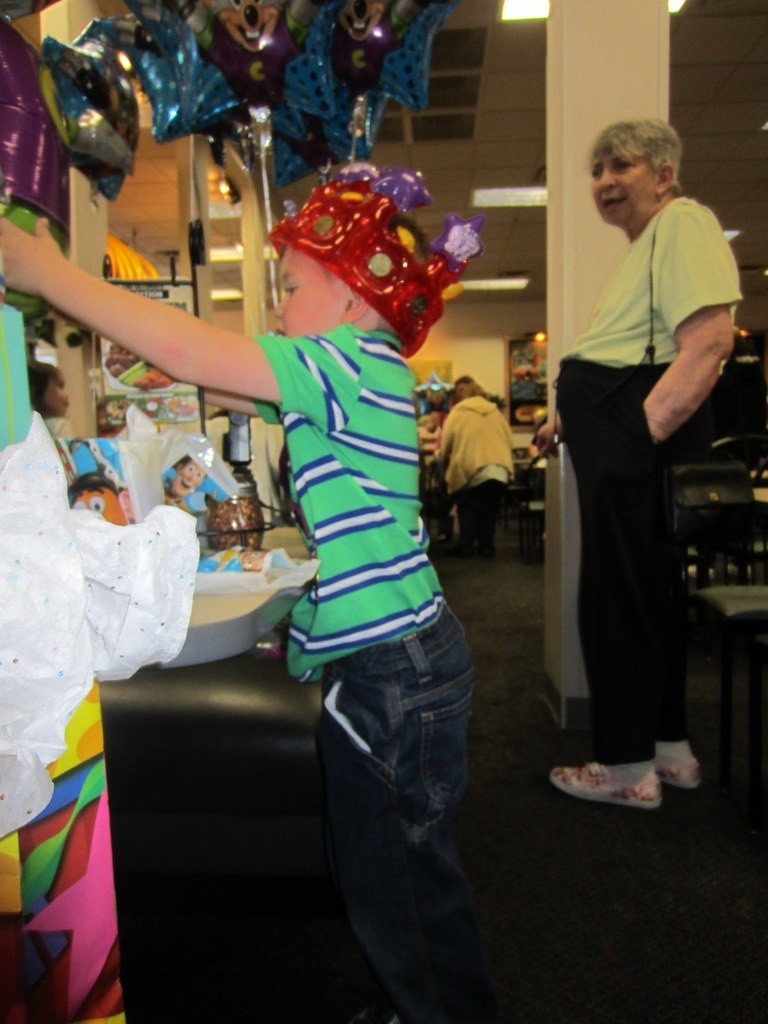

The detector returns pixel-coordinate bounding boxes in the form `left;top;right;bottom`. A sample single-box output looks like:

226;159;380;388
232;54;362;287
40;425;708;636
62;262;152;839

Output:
207;471;265;552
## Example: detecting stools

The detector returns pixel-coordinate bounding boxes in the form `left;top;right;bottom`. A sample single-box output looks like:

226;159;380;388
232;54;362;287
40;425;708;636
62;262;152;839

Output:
507;455;768;830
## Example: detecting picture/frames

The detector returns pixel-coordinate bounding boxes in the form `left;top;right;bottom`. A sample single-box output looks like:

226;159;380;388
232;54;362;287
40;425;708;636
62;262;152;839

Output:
505;333;548;432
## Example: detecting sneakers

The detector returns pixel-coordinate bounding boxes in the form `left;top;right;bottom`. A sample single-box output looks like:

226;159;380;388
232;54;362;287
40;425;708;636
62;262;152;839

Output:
551;759;666;808
655;760;708;791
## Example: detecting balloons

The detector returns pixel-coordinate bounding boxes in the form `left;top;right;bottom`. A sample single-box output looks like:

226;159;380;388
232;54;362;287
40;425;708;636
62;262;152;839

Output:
333;162;487;272
41;0;458;200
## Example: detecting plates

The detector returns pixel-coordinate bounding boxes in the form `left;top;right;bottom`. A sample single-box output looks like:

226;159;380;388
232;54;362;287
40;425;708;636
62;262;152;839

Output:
165;396;197;420
102;358;179;392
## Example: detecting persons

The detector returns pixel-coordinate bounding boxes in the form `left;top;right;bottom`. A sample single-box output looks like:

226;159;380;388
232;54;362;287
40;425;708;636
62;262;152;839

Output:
417;376;516;558
0;182;497;1024
533;120;743;808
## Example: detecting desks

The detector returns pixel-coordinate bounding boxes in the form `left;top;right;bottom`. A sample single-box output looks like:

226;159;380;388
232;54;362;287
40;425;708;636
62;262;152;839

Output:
100;668;394;1024
513;457;547;566
